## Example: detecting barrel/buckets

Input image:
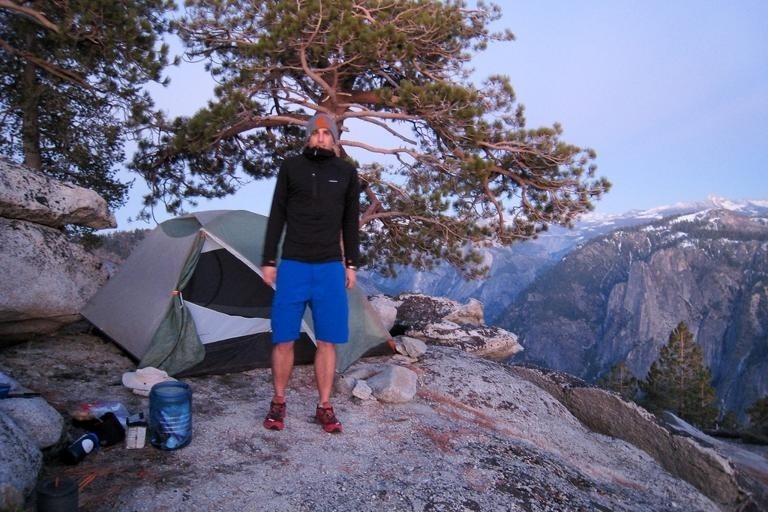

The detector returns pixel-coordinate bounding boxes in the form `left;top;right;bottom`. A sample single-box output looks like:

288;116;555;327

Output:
148;382;192;449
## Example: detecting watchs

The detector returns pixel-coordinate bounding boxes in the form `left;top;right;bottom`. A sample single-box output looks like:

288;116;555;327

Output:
346;265;357;271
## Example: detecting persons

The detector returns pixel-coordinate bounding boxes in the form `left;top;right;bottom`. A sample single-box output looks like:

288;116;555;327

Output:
257;112;368;433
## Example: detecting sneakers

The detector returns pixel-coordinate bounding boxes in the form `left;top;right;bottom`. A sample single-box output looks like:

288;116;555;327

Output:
316;402;343;433
263;401;287;431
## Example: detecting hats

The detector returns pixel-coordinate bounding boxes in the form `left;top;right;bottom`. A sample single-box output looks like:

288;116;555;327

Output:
306;113;339;145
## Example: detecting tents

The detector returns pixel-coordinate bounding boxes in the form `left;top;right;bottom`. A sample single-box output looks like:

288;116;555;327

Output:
76;206;394;382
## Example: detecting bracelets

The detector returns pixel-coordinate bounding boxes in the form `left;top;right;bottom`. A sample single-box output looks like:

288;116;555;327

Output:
262;263;275;266
